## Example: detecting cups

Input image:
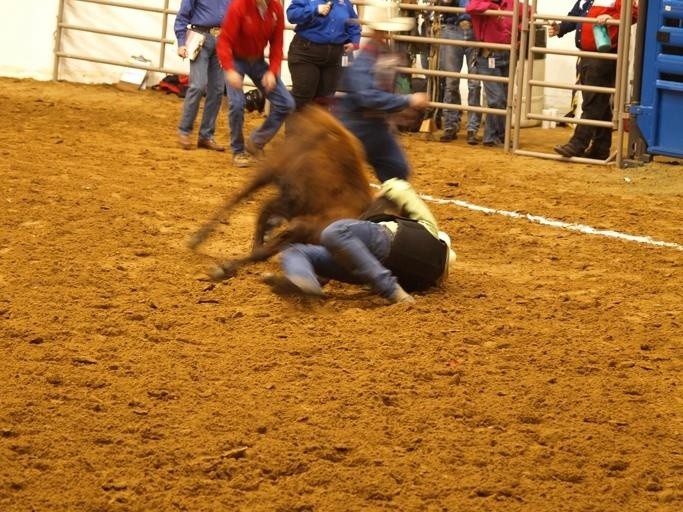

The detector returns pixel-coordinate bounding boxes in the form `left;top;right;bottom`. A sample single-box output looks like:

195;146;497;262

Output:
592;24;611;52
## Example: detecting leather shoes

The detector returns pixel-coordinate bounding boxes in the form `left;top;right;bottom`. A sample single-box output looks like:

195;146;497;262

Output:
584;140;611;161
176;130;197;151
553;138;586;158
197;138;226;152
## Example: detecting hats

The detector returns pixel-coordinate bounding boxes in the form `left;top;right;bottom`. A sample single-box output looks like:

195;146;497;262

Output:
344;1;416;34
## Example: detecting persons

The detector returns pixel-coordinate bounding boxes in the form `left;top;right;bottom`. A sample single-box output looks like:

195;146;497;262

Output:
259;178;447;305
173;0;531;183
548;0;638;160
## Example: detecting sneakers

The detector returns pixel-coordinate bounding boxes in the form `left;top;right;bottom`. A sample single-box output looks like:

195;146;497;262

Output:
245;138;268;162
230;149;252;168
483;140;521;151
467;130;481;144
440;129;459;143
389;283;418;308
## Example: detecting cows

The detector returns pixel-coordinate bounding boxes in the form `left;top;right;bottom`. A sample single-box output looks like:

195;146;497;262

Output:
185;103;375;285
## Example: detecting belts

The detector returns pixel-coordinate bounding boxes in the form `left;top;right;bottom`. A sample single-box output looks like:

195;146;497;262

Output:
190;24;222;38
446;20;472;31
